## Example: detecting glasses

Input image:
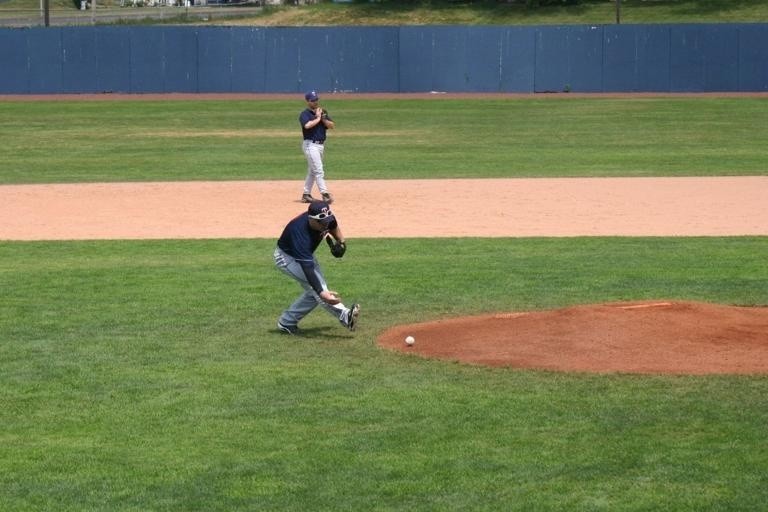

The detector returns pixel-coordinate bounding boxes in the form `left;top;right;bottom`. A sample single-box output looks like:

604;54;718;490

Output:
309;210;332;220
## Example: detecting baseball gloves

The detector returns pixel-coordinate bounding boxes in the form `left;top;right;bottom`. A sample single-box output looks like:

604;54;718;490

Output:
326;235;346;256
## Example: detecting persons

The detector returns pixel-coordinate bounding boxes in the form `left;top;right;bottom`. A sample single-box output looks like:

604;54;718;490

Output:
273;199;360;338
297;90;335;207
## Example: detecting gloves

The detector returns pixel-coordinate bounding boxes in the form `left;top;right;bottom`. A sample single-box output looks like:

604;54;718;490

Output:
331;243;346;258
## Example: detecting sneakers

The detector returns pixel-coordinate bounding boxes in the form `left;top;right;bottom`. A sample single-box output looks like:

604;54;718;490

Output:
348;304;360;331
322;192;331;203
278;321;304;336
302;194;315;203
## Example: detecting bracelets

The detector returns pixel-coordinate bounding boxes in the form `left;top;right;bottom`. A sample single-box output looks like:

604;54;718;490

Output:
315;115;321;118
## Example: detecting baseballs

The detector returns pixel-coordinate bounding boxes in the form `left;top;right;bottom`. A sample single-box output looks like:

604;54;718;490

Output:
406;336;414;345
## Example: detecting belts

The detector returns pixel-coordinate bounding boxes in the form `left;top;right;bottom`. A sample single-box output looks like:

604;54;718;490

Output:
305;139;324;143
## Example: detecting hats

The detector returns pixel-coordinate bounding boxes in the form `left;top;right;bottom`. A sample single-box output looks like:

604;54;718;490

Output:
305;91;319;100
308;201;335;222
